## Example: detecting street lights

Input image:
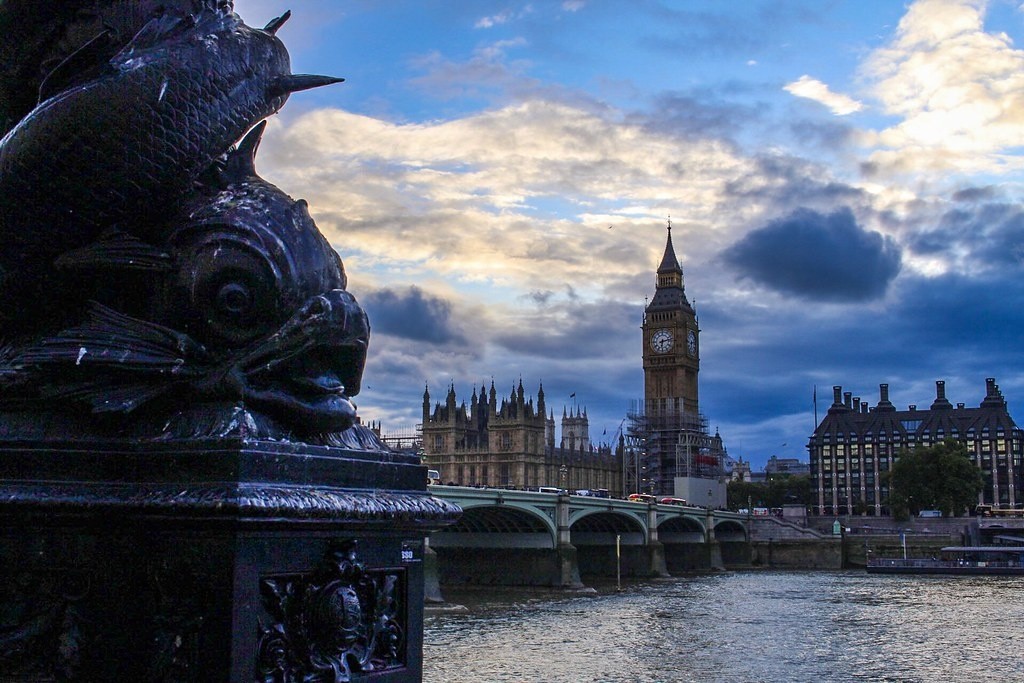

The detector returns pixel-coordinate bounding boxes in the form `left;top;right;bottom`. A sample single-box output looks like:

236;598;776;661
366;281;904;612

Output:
768;478;774;514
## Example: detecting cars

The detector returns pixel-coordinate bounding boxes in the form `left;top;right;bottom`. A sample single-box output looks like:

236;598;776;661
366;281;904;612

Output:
427;469;440;486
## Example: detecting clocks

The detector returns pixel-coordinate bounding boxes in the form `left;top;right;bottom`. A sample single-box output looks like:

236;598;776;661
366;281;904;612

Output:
652;330;673;353
687;331;697;355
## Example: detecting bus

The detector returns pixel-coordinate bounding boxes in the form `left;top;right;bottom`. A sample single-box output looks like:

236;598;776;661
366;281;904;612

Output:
628;493;657;503
919;510;943;518
661;497;686;505
538;486;569;494
